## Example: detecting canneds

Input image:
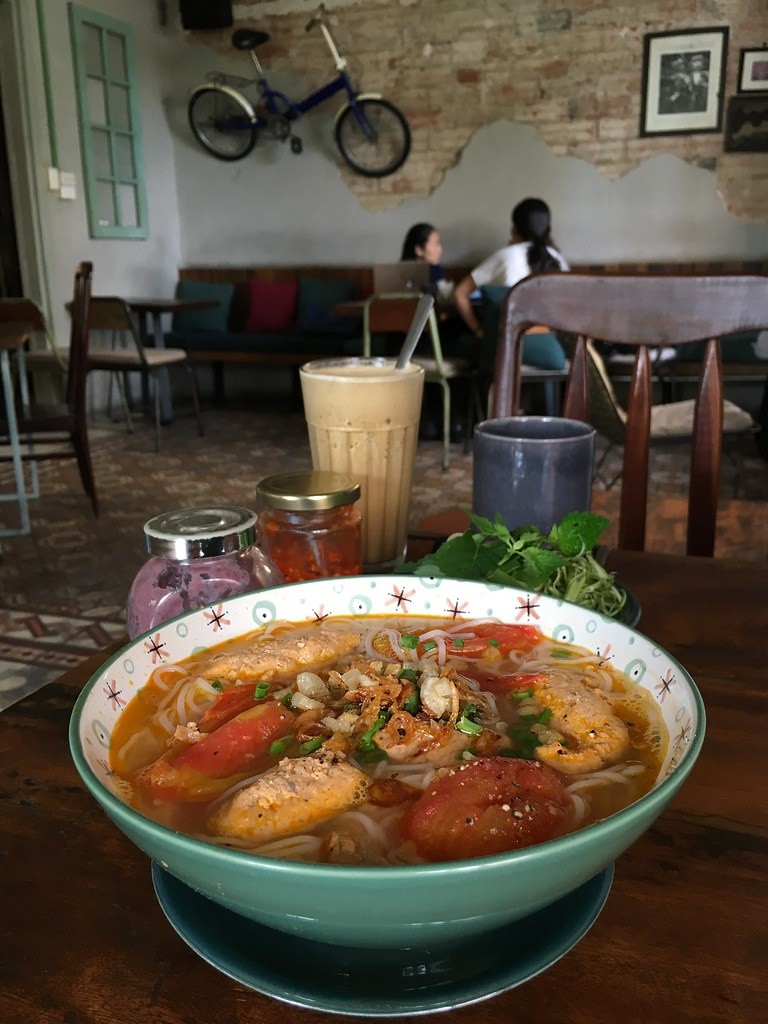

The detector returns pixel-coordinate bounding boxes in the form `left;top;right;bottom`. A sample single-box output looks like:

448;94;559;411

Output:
125;469;360;638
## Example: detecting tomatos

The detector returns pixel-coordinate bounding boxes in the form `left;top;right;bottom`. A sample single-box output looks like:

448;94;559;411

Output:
404;757;569;860
137;701;300;801
414;623;541;662
455;670;547;689
204;681;285;729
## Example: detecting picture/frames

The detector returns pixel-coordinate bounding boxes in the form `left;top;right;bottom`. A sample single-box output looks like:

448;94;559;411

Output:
724;94;768;152
737;47;768;92
639;25;728;137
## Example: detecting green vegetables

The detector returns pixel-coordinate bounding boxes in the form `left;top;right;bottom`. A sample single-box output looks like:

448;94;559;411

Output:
396;507;631;623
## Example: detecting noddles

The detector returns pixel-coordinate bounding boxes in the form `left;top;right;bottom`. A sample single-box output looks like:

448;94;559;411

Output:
108;613;668;866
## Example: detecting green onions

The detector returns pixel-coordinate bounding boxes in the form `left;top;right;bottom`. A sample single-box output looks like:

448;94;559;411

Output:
210;634;571;760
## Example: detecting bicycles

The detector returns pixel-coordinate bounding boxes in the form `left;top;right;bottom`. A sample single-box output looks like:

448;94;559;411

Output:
184;3;413;180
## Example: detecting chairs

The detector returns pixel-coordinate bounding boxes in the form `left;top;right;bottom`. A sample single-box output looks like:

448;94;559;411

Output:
362;273;768;560
0;261;203;517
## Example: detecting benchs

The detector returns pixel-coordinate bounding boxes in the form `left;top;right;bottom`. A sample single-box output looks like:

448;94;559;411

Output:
172;260;768;431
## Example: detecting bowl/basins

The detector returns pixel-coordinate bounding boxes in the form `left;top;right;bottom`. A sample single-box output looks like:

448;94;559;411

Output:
70;576;707;951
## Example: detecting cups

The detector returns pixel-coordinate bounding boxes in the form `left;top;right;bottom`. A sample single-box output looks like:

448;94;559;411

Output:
471;417;595;533
300;357;425;571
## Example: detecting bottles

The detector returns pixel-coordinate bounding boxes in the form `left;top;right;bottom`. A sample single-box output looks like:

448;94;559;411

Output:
255;470;363;586
127;504;287;642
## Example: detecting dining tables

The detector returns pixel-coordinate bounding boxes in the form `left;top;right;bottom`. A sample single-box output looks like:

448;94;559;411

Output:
1;532;768;1021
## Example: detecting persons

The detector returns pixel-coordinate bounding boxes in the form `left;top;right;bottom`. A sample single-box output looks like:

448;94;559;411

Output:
455;196;570;442
369;222;464;443
666;55;709;112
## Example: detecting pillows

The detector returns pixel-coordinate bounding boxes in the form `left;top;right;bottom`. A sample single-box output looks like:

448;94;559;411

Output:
243;279;296;334
299;276;356;324
175;279;234;336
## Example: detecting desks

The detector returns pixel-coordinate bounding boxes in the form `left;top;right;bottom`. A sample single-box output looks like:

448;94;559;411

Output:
0;296;46;539
106;297;220;425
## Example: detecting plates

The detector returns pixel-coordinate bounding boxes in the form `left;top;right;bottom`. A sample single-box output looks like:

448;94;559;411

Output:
613;582;641;627
151;861;615;1017
